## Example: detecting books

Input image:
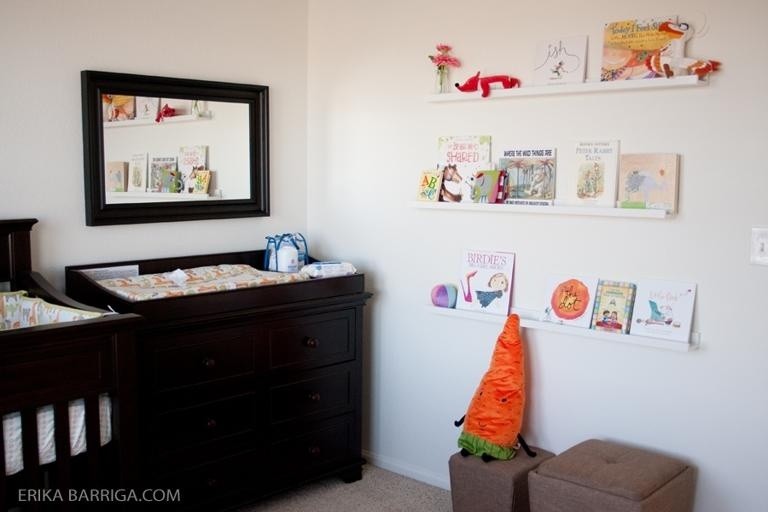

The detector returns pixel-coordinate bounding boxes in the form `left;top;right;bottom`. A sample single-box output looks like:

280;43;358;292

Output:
598;13;679;81
416;131;681;215
103;94;196;122
104;143;213;195
540;273;698;344
532;35;588;86
453;249;515;316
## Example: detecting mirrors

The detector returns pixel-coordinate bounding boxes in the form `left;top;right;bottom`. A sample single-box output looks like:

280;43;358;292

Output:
81;70;270;226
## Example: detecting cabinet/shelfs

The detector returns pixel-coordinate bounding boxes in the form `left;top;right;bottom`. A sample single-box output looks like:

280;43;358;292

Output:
102;111;222;200
411;75;709;352
66;250;373;512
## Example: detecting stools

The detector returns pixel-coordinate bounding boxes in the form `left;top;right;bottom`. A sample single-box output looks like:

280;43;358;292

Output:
528;440;696;512
448;444;554;512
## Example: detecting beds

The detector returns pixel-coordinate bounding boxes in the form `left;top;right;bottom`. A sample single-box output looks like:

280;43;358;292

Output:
0;218;145;512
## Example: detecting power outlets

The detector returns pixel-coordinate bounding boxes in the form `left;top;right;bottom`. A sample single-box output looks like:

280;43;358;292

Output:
751;227;768;265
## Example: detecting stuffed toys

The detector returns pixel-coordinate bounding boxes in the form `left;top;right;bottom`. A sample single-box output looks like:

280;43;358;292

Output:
454;314;538;464
431;282;458;308
644;16;720;82
454;71;521;97
